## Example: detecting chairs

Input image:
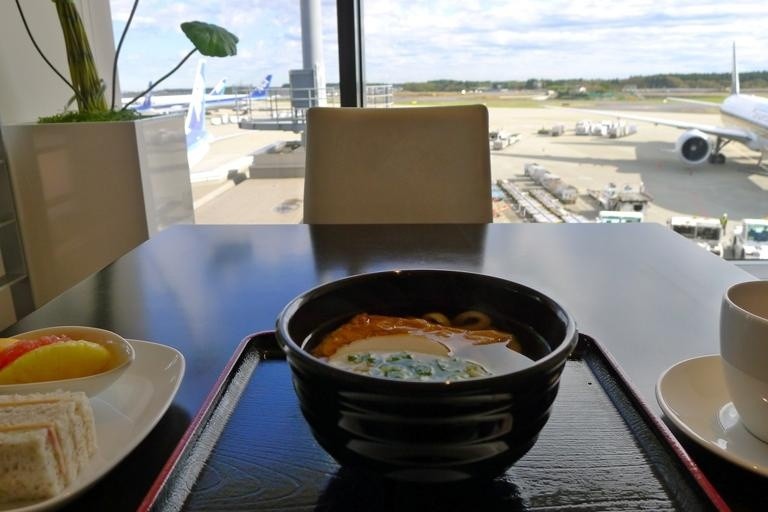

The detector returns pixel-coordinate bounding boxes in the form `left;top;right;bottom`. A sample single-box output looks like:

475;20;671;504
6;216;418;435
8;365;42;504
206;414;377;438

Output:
303;104;492;222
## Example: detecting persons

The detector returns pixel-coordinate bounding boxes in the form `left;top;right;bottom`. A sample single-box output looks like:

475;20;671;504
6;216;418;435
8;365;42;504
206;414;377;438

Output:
720;213;728;236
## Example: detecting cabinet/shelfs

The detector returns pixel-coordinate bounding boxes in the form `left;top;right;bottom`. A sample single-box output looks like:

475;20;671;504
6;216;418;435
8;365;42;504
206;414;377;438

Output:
0;132;36;334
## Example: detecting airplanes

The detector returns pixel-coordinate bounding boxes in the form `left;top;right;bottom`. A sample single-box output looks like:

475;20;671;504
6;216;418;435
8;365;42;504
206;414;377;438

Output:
121;64;270;171
547;40;767;167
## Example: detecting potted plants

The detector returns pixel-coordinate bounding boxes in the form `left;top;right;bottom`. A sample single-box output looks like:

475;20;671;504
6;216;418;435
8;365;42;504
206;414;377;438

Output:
0;0;239;307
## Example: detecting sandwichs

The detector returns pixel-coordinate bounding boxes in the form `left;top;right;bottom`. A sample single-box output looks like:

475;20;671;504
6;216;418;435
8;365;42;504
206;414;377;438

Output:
0;389;97;505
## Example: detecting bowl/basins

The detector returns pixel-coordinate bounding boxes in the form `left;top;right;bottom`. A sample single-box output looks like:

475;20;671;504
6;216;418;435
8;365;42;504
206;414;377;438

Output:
716;276;768;447
272;265;583;486
0;322;137;400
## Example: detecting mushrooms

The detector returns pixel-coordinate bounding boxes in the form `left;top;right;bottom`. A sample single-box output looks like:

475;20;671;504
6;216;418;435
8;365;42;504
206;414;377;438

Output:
423;310;491;331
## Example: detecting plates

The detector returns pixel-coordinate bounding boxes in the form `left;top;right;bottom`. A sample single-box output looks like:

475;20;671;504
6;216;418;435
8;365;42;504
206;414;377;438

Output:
0;336;191;512
653;352;768;478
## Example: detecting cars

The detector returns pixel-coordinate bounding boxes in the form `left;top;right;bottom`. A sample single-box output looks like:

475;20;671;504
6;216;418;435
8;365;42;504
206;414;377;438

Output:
732;218;767;260
